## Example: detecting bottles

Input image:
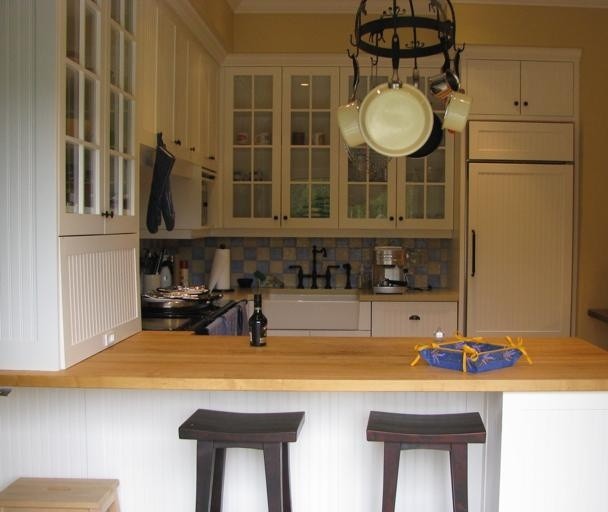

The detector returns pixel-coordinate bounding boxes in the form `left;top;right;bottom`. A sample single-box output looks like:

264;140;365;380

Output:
159;265;173;289
179;260;189;287
247;292;268;347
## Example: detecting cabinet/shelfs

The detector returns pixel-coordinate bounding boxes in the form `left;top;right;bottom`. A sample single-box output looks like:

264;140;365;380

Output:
456;39;584;115
221;49;335;239
141;2;226;178
246;292;371;337
461;160;579;338
337;49;455;239
2;0;141;237
1;236;146;371
460;114;581;161
371;298;458;337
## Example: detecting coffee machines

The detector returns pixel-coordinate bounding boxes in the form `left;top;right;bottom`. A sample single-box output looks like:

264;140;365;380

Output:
369;242;409;294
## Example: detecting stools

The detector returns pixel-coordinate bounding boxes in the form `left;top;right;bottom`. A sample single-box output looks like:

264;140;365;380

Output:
178;408;305;511
0;478;120;512
366;410;486;512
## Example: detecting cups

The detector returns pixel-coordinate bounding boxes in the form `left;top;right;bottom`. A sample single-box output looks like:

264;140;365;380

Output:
232;122;330;147
144;273;160;290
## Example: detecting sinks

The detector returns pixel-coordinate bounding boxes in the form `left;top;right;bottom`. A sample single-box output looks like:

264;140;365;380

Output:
267;294;359;330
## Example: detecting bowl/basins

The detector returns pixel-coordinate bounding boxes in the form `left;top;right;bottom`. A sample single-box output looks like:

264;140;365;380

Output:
237;277;253;288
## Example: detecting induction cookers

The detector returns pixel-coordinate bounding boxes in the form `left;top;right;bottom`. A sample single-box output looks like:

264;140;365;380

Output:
142;296;241;331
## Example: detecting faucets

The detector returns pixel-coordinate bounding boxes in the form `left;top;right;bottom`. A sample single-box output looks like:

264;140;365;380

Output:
311;245;327;288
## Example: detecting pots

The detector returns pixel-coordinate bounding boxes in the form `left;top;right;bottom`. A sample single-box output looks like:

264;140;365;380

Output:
141;283;224;315
334;27;473;161
